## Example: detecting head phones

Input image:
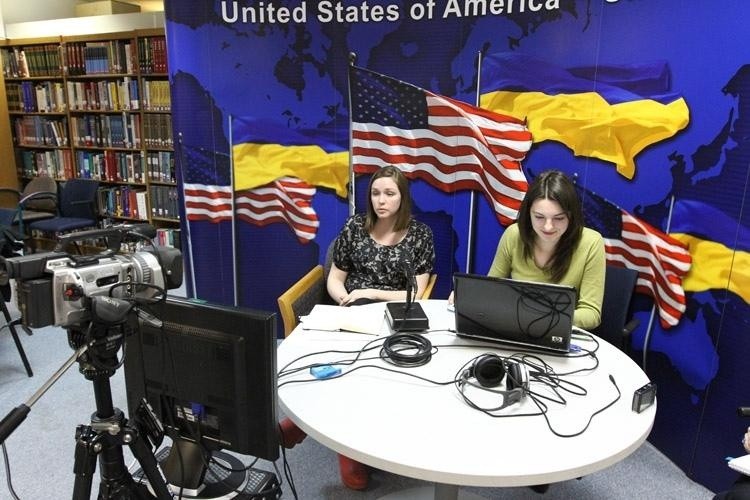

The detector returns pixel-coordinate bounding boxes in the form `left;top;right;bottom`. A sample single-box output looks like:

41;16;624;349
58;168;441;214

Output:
458;353;530;412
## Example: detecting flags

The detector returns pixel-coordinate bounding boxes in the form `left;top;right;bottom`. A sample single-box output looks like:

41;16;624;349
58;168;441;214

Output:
348;64;750;334
179;136;349;243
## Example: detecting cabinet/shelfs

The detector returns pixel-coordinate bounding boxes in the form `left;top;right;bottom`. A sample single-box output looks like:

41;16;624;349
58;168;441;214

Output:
0;27;180;248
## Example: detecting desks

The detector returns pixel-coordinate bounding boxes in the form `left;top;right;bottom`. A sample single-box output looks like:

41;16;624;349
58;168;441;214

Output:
277;300;658;500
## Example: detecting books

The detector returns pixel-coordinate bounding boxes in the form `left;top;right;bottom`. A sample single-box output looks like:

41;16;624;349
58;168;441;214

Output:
1;36;182;251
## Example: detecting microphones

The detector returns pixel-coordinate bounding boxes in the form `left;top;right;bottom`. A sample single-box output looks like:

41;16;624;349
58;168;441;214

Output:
385;255;431;331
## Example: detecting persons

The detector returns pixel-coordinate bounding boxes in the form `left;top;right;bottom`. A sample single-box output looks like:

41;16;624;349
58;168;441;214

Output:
447;170;606;497
278;165;435;493
712;426;750;500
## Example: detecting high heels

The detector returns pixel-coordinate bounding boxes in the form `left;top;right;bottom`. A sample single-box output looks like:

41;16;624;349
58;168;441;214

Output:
339;455;369;489
279;418;306;448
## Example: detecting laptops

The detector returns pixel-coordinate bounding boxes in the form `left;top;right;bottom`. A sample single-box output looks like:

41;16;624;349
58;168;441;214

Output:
453;272;576;353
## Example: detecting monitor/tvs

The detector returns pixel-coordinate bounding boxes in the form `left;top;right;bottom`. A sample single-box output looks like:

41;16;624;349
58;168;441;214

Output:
121;293;277;500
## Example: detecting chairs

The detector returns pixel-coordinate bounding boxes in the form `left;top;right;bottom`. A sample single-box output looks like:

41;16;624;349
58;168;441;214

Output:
590;267;639;347
0;175;58;227
19;176;100;244
278;265;438;338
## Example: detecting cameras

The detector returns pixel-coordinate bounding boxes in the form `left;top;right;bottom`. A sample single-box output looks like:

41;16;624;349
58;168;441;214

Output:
631;382;657;413
